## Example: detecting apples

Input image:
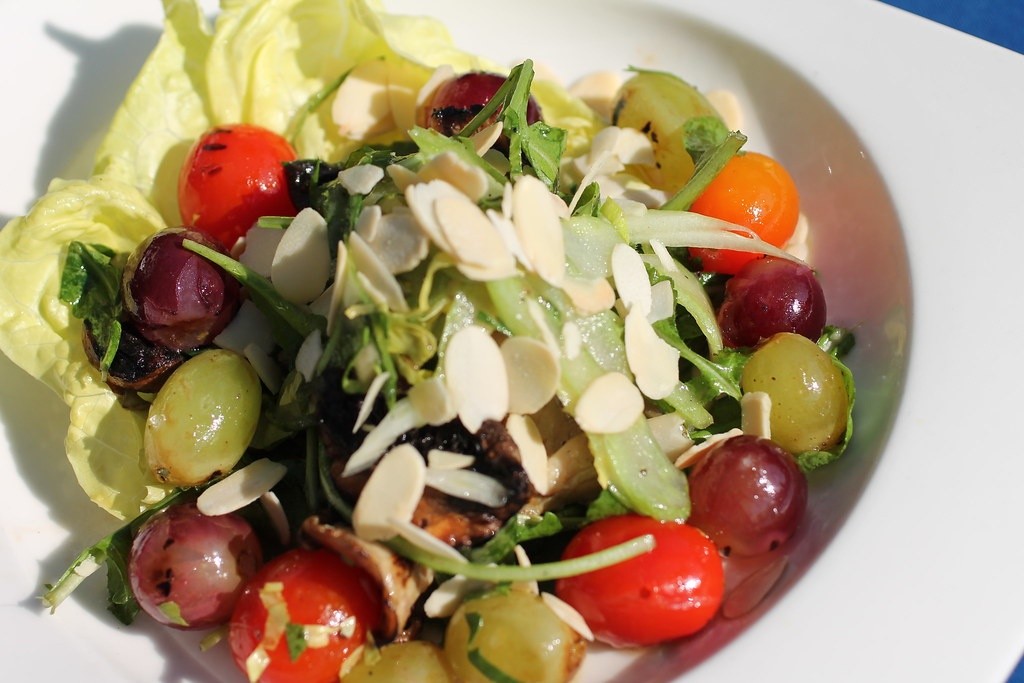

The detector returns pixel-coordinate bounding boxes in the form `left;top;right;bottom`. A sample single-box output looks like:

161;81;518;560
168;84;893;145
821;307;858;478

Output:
340;638;459;683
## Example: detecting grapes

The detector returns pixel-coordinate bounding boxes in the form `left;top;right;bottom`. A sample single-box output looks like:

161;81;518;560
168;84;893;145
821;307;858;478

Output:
743;333;846;458
143;349;264;487
443;590;589;683
712;256;827;349
426;72;544;161
127;498;288;630
684;432;807;555
121;228;239;349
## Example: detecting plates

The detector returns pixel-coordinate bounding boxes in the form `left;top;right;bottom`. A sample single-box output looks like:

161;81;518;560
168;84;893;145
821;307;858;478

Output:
128;0;916;682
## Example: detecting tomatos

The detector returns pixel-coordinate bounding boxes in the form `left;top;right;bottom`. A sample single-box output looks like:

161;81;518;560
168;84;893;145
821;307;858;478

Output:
552;515;722;647
177;123;299;253
226;545;396;683
683;151;802;273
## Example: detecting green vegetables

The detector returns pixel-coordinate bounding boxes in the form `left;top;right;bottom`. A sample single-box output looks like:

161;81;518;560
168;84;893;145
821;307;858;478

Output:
33;58;856;623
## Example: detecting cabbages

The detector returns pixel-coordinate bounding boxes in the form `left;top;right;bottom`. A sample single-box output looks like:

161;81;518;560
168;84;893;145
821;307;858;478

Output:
0;0;621;531
